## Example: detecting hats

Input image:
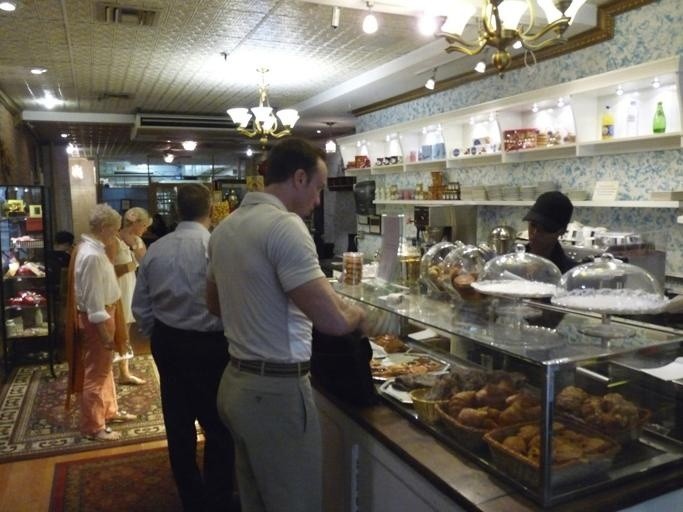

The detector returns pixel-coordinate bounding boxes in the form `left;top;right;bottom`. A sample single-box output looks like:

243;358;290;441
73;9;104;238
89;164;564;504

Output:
522;191;573;233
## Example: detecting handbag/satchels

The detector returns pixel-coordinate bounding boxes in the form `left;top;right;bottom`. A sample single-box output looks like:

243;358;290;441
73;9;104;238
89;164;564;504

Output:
309;321;380;407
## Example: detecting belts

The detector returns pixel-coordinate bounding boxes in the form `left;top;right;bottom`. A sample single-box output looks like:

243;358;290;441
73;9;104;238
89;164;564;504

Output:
227;355;310;379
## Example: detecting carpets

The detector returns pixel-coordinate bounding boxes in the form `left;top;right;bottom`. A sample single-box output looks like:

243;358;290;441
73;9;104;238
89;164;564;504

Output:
0;350;202;464
43;441;240;511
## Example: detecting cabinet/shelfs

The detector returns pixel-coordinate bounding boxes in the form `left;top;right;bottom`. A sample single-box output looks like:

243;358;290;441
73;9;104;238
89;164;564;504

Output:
308;273;683;512
338;56;682;212
0;186;60;382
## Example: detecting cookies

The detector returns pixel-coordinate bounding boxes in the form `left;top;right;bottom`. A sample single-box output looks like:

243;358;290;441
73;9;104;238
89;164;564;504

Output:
344;258;362;286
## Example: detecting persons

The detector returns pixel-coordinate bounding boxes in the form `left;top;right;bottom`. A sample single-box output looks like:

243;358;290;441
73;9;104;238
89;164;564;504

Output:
206;137;368;511
117;206;155;387
131;185;234;511
72;202;138;441
512;192;572;281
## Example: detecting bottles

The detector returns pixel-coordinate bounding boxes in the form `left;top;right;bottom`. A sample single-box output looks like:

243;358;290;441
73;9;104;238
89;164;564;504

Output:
5;320;16;337
440;244;496;304
34;309;42;326
624;100;639;137
652;101;667;134
341;251;363;291
600;107;614;141
416;239;466;295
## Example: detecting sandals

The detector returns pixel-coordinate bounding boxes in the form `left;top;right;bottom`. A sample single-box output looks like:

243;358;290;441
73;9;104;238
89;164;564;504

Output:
105;410;137;425
80;426;121;442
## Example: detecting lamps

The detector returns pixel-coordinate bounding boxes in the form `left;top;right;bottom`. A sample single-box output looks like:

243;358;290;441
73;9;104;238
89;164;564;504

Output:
161;148;176;165
430;0;585;79
424;68;438;93
223;67;301;150
361;0;377;34
324;120;337;154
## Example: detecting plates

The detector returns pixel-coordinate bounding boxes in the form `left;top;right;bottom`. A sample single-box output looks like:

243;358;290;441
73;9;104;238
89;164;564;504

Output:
647;190;682;201
460;179;587;202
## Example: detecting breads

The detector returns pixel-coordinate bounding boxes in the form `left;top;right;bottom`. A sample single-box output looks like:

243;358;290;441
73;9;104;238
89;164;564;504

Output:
429;261;486;301
367;328;639;468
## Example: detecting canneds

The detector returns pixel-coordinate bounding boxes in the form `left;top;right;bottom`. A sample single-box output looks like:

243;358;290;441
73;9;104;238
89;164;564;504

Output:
414;172;460;200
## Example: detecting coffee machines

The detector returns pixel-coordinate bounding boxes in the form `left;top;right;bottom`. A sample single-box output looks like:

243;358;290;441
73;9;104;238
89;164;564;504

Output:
412;206;457;254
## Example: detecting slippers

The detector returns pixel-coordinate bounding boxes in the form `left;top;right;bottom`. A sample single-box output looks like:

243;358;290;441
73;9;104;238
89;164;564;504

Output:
118;376;147;385
